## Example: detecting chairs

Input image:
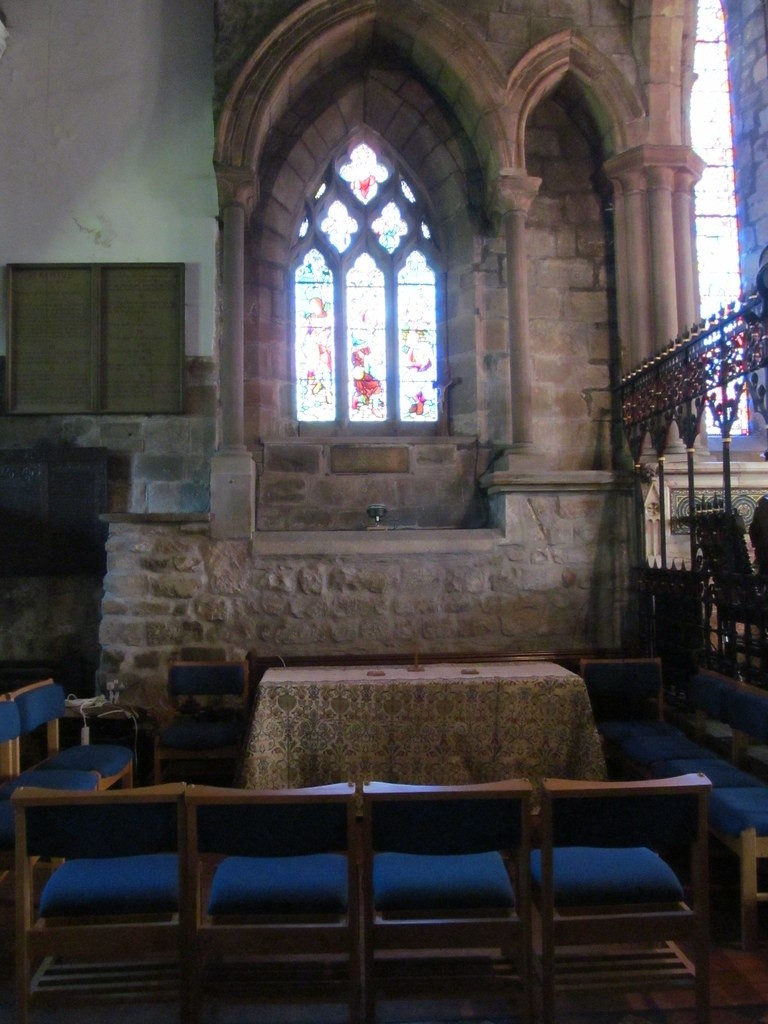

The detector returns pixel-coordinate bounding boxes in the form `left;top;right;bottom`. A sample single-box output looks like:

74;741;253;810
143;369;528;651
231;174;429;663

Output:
0;664;768;1024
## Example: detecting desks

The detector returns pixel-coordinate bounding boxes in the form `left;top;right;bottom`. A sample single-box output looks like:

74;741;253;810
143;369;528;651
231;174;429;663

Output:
242;662;608;815
